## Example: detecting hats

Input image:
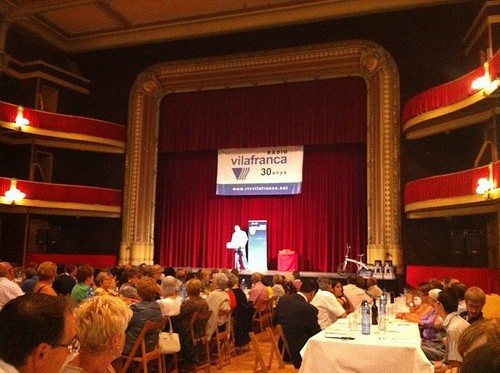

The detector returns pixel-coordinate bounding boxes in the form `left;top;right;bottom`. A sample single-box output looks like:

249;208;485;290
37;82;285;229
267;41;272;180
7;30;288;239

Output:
429;289;442;302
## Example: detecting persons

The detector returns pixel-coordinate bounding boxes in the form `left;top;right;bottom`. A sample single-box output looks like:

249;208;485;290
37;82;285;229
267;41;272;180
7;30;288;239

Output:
356;276;373;298
457;318;500;373
271;280;322;369
308;276;347;331
0;261;303;373
331;279;354;315
396;278;487;373
366;277;383;297
230;224;249;271
0;292;79;373
342;274;371;309
61;294;134;373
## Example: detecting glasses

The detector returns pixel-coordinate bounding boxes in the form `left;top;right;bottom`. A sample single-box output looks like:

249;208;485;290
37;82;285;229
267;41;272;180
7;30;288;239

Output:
47;338;81;355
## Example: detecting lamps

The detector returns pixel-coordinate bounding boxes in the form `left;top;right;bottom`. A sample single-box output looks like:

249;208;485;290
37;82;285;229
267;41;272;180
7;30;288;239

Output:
475;177;495;200
3;189;27;204
11;114;30;131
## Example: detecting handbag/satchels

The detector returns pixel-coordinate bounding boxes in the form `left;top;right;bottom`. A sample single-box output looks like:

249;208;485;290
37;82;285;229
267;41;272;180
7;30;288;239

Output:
157;332;181;355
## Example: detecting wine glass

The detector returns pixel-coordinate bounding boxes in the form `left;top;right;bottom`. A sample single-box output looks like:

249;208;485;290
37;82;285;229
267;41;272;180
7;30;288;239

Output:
378;323;386;340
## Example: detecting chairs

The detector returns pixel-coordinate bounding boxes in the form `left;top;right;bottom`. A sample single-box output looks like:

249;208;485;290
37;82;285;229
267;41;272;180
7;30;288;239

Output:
110;293;302;373
374;259;383;274
383;260;394;275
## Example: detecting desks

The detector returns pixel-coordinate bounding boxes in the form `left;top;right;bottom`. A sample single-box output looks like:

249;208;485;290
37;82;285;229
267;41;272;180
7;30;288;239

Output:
296;302;437;373
277;253;299;271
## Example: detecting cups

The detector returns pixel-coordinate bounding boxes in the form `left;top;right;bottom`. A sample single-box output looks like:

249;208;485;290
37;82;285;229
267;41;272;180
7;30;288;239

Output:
348;313;356;332
387;296;407;323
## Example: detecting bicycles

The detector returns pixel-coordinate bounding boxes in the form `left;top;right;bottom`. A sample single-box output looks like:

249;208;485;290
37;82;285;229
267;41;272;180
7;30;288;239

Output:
336;244;376;280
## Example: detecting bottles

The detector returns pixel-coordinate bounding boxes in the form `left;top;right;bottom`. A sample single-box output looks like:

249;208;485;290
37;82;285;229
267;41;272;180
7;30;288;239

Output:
372;298;378;325
361;300;370;335
